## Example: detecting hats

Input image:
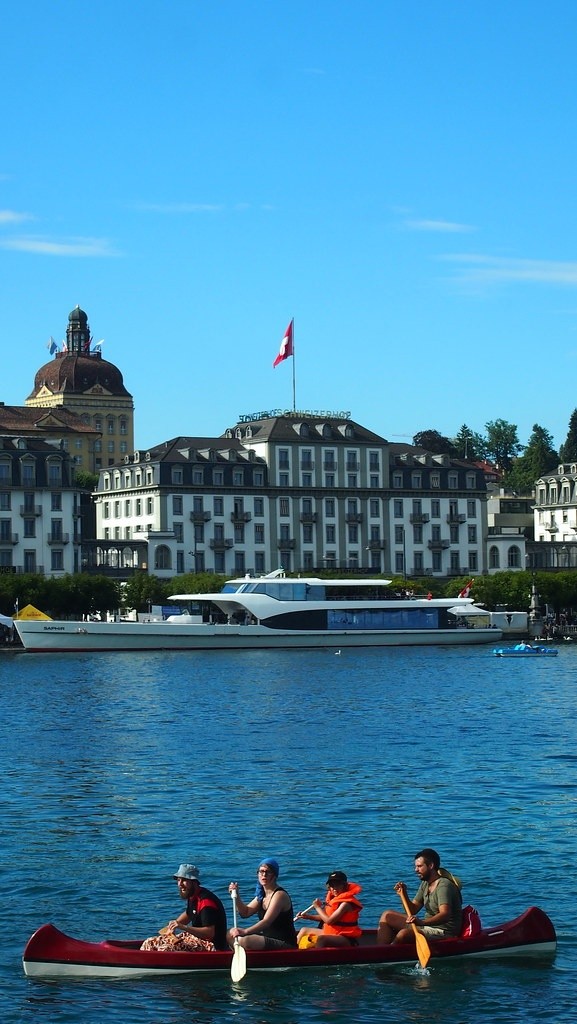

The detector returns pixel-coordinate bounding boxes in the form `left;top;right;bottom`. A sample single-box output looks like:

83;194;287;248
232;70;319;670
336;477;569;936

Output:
437;867;463;905
173;864;201;885
325;872;348;885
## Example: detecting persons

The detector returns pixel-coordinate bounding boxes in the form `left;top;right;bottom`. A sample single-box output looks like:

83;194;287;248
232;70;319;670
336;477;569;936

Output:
545;615;567;640
140;849;465;952
143;620;150;622
0;626;17;644
520;640;532;648
450;616;497;629
401;588;415;600
126;564;128;567
327;585;389;600
203;611;245;624
110;620;112;622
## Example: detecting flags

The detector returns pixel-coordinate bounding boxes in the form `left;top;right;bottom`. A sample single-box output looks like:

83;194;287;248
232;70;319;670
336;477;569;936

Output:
46;336;105;355
273;319;294;369
457;578;475;598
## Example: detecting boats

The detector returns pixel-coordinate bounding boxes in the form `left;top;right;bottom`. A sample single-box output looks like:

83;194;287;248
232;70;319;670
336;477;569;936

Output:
13;563;504;654
492;640;559;657
21;905;557;980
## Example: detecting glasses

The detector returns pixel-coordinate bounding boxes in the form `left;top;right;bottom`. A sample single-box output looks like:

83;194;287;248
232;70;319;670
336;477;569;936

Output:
258;870;274;876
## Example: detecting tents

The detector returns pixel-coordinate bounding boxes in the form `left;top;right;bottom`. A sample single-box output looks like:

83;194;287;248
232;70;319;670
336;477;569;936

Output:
11;604;53;620
0;613;13;628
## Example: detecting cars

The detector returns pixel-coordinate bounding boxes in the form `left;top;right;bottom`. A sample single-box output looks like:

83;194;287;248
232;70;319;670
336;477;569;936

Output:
0;614;15;644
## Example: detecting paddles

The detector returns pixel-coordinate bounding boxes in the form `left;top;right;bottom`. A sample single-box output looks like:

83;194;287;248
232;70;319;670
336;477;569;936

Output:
292;900;316;923
228;882;246;982
394;883;430;968
